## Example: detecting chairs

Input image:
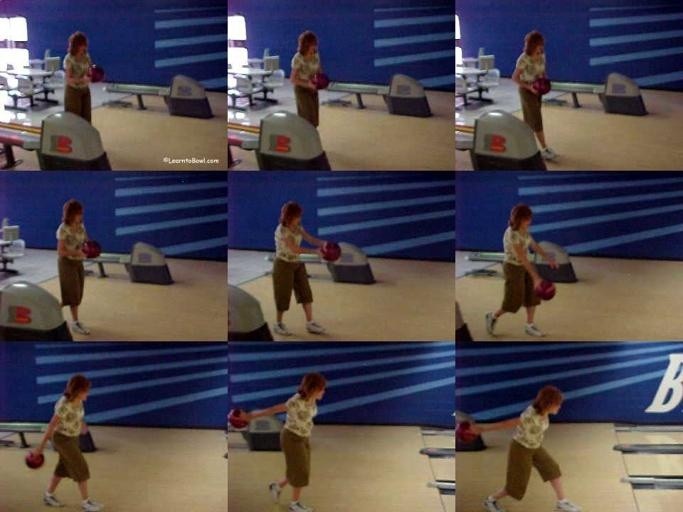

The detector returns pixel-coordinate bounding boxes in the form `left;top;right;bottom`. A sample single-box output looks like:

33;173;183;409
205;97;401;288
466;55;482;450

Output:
455;55;501;106
0;219;25;273
228;62;285;111
0;60;64;111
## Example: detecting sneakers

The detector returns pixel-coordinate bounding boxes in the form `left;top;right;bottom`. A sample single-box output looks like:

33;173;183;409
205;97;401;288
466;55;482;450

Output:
485;311;496;334
556;501;582;512
306;322;324;333
268;480;283;503
72;322;89;335
290;502;314;512
543;147;555;159
482;496;505;512
81;499;103;511
525;325;546;337
272;322;291;336
43;495;64;507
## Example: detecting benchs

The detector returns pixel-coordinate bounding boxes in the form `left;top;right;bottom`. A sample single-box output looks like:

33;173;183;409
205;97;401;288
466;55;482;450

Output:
227;130;260;168
103;84;171;109
328;82;388;108
259;256;322;276
455;132;474;151
465;251;535;276
0;122;43;169
546;82;606;106
0;423;46;448
85;255;120;276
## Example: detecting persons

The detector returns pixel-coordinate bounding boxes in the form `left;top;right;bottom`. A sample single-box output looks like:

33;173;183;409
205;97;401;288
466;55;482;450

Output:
28;375;104;511
511;30;564;163
484;203;558;336
233;372;327;512
467;385;580;511
61;29;92;125
272;201;327;334
53;198;92;335
289;30;323;127
